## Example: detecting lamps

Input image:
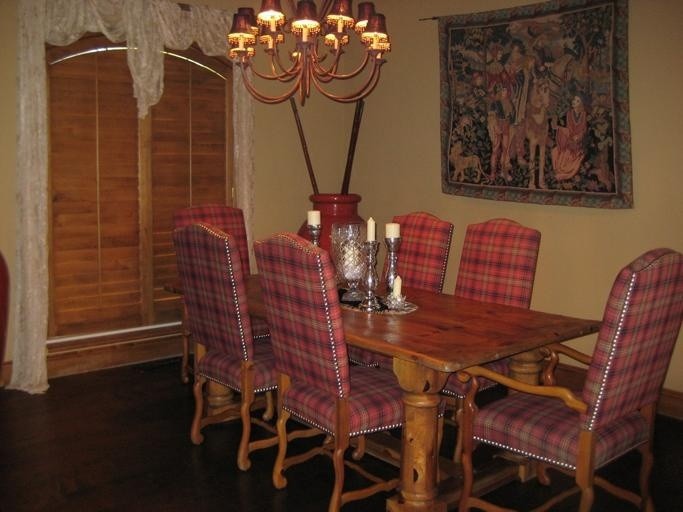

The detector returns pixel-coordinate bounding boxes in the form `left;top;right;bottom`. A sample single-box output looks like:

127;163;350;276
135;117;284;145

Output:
226;0;392;107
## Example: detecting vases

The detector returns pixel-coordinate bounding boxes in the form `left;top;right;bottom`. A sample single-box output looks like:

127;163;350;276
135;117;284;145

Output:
295;194;367;283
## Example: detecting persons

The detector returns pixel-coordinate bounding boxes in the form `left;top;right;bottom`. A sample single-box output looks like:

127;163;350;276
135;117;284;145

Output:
482;41;611;190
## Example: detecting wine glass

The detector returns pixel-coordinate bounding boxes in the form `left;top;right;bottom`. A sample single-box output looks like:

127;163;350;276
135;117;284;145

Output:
330;222;370;304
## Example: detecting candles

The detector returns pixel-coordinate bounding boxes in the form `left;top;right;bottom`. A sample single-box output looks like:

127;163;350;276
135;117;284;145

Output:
306;210;321;228
366;216;376;242
385;223;401;238
392;275;402;299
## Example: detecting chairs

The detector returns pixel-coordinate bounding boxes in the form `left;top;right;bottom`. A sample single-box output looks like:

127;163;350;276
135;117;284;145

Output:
171;221;335;472
344;211;454;368
252;231;447;512
454;246;683;512
438;218;542;464
170;202;271;384
